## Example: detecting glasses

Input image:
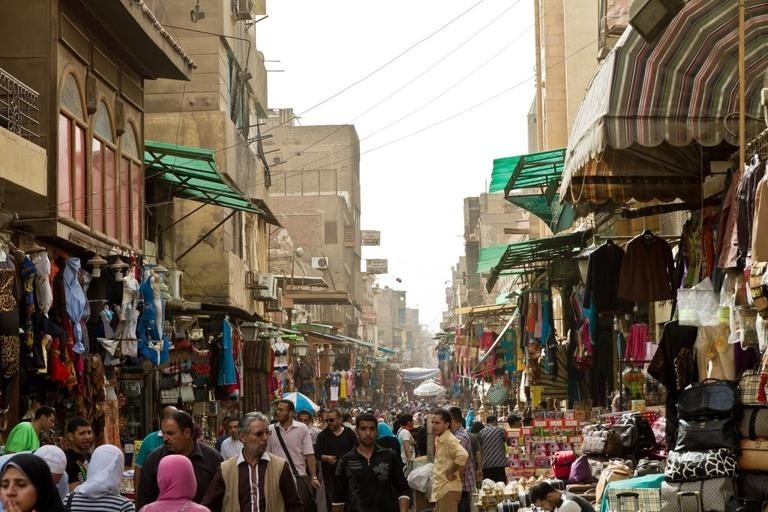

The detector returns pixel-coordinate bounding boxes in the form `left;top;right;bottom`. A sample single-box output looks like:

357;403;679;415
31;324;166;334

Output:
247;429;272;436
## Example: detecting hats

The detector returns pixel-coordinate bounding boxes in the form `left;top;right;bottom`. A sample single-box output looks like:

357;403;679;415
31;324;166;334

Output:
508;414;522;423
34;445;68;475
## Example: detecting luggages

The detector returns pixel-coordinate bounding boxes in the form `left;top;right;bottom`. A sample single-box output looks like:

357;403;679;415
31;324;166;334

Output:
566;483;596;511
497;479;564;512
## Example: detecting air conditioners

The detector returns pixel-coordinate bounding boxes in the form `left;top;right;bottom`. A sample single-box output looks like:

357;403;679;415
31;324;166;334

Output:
268;288;282;312
237;0;255;20
168;270;184;301
311;256;328;269
259;275;277;299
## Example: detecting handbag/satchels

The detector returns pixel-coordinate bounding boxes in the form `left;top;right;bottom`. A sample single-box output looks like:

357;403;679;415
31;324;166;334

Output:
297;475;319;512
581;413;655;455
596;459;660;503
568;456;593;484
661;369;768;511
551;450;576;478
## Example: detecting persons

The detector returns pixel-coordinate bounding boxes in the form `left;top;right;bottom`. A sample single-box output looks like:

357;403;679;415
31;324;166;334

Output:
118;265;163;356
331;369;353;397
539;401;548;412
215;400;322;512
133;406;225;511
217;314;244;398
523;417;532;426
428;401;511;511
270;337;288;375
315;403;427;511
529;482;595;511
508;414;521;428
1;401;135;511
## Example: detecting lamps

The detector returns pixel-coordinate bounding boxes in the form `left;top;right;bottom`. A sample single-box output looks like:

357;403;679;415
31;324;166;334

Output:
73;234;129;281
0;226;46;262
161;24;252;84
190;0;205;23
629;0;684;44
